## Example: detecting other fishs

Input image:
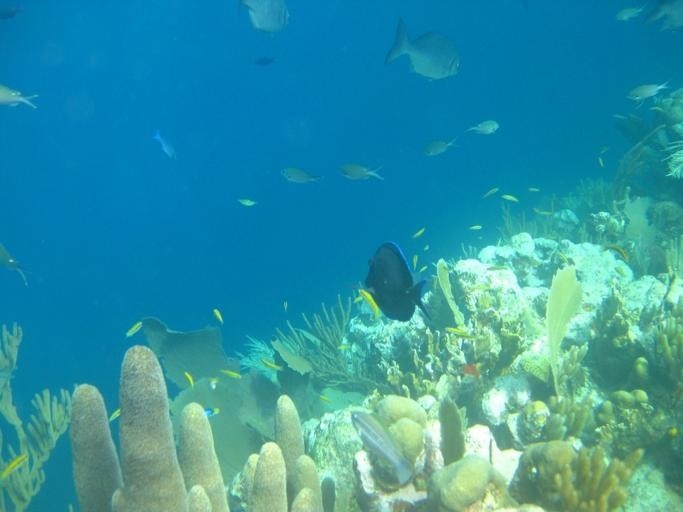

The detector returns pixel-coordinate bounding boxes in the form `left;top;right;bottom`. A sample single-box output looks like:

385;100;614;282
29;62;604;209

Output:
211;308;225;331
352;223;430;323
596;243;627;263
277;163;387;187
626;79;677;100
468;187;557;250
318;344;354;406
120;321;144;340
380;21;459;82
241;2;294;36
111;358;285;423
595;112;642;172
425;119;500;158
235;191;258;212
0;1;682;511
2;85;41;111
441;325;480;381
1;453;29;484
614;2;640;24
